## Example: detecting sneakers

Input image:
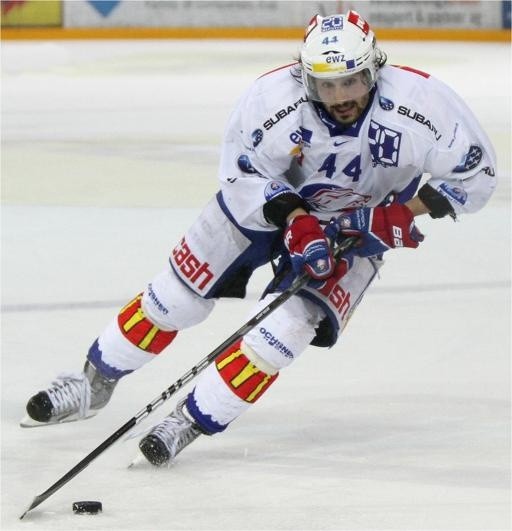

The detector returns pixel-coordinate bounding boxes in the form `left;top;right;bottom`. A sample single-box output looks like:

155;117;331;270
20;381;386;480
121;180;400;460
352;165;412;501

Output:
138;396;204;468
27;361;119;424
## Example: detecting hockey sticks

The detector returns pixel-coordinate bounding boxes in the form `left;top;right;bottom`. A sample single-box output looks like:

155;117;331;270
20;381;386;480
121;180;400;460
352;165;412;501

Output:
20;238;358;520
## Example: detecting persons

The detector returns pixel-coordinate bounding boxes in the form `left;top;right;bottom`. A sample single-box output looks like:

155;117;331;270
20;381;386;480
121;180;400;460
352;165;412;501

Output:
25;11;498;467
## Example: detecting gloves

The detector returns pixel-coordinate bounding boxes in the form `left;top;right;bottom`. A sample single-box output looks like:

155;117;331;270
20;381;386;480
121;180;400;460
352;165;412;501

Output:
283;203;425;290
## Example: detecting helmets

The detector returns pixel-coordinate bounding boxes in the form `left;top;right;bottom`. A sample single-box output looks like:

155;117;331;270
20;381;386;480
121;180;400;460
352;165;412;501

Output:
301;10;387;103
